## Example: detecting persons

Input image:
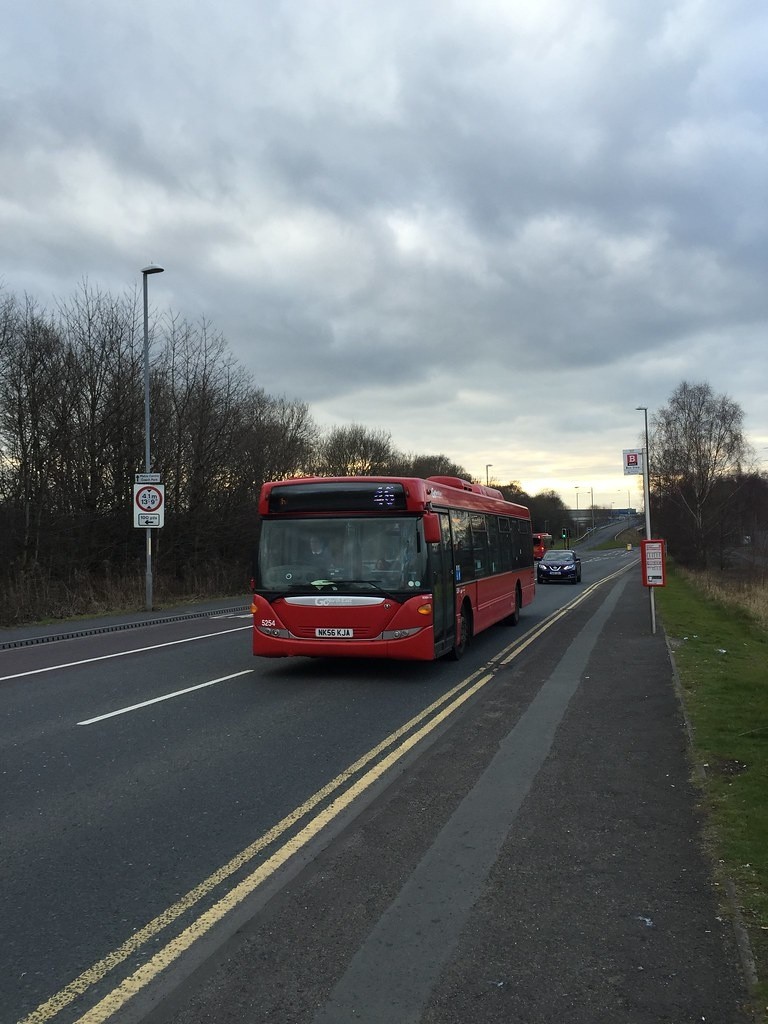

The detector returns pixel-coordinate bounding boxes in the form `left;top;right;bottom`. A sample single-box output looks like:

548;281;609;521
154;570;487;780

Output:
301;535;337;570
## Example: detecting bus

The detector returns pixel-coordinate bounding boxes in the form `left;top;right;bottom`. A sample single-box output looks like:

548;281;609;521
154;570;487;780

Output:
251;476;536;659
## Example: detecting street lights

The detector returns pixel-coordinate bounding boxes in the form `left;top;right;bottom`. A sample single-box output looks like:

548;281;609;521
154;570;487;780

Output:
618;489;630;526
576;492;590;538
486;465;493;487
611;502;614;518
575;486;594;529
142;260;165;609
635;404;654;542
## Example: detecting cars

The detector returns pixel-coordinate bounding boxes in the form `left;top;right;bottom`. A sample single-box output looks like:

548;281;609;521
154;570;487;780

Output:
533;533;553;560
537;550;581;584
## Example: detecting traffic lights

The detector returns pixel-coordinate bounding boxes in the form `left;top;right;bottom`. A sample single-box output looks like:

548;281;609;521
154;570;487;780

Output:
562;528;566;539
